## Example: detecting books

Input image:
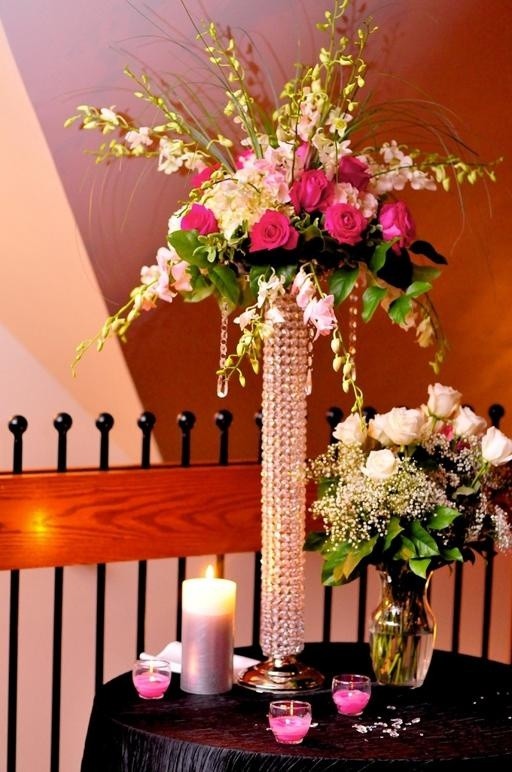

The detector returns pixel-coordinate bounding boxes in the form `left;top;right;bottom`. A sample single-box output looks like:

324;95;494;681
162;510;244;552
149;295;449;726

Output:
140;642;260;675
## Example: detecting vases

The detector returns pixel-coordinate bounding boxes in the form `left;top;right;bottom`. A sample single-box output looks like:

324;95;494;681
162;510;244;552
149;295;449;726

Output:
227;275;331;699
366;553;435;690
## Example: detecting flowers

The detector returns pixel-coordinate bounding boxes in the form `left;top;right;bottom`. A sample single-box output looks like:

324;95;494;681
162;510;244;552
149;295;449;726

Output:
57;2;507;423
312;383;512;582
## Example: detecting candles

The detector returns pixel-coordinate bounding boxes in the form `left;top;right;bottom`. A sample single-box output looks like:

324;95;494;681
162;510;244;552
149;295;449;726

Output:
179;562;241;694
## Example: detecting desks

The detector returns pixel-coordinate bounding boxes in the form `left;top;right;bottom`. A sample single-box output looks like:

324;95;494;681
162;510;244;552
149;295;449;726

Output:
81;641;512;768
